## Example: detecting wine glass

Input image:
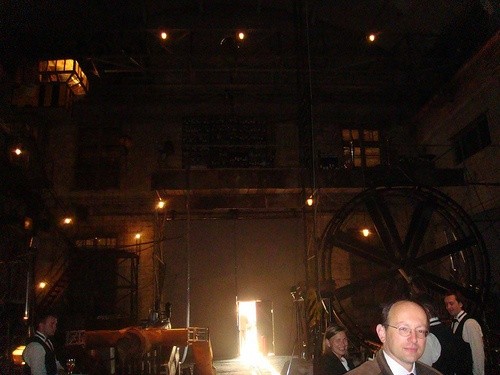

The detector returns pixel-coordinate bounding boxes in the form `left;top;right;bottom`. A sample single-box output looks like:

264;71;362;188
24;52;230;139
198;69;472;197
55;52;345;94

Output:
66;356;76;375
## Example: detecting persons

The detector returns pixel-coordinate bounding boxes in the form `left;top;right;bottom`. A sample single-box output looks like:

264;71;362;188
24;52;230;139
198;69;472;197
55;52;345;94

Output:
319;323;355;375
342;297;444;375
23;309;67;375
418;332;441;367
442;287;485;375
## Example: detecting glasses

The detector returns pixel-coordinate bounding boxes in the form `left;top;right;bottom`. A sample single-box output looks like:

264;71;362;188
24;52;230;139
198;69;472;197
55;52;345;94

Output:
387;324;426;340
